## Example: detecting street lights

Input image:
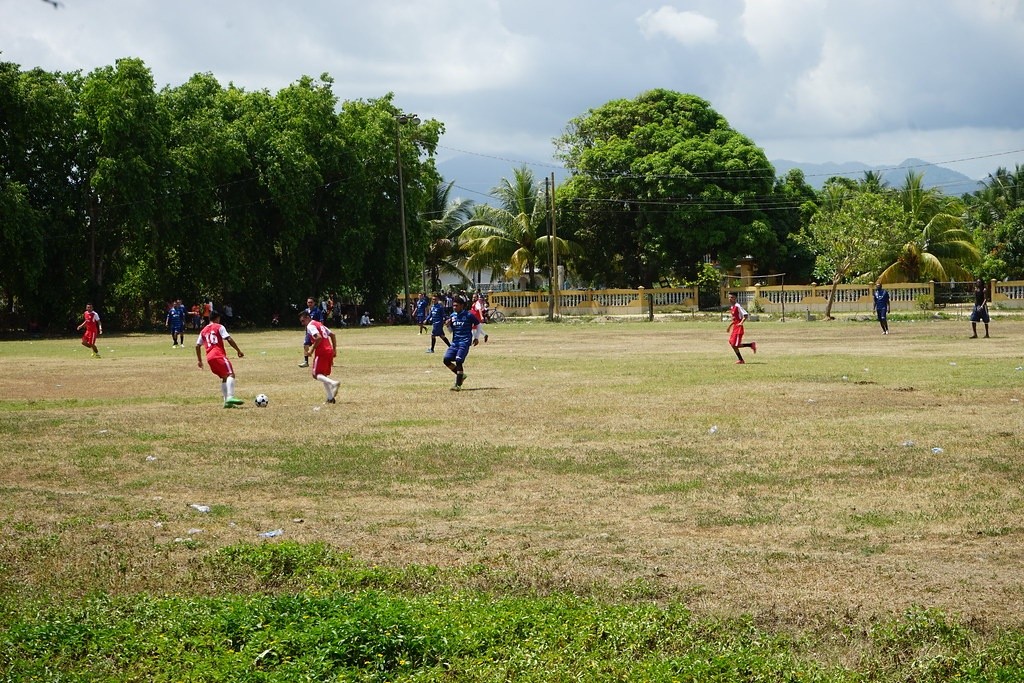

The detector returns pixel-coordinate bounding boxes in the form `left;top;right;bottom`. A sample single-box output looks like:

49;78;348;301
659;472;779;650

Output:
395;113;421;326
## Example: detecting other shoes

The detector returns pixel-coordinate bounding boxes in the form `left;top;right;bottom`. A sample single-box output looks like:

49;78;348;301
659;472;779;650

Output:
426;349;434;353
173;345;177;349
91;352;101;359
298;363;309;367
425;326;429;333
418;332;422;336
450;385;461;391
484;335;488;342
180;344;185;348
462;373;468;383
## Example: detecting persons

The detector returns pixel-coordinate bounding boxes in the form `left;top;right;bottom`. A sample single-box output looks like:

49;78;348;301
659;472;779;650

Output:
298;297;334;367
726;292;756;364
165;299;214;347
223;302;234;330
271;315;279;328
969;278;990;339
298;312;340;404
872;284;890;335
386;301;407;325
410;288;488;354
196;311;245;408
359;311;371;327
319;293;347;328
443;298;480;391
77;303;103;358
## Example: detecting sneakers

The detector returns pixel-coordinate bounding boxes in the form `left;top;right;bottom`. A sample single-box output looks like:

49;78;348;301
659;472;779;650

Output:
323;398;336;404
224;402;238;408
225;395;244;405
331;381;340;397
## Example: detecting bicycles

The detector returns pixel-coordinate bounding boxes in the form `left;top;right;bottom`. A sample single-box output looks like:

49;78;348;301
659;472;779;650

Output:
482;306;506;324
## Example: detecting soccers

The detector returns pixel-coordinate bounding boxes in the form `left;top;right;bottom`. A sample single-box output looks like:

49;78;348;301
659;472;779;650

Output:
255;394;269;408
